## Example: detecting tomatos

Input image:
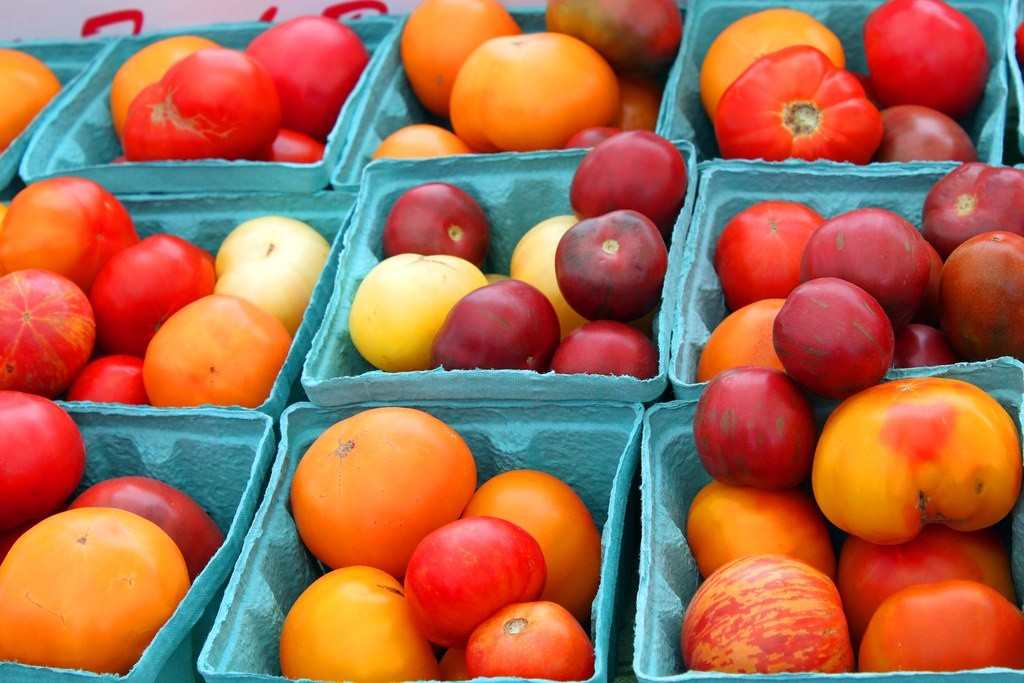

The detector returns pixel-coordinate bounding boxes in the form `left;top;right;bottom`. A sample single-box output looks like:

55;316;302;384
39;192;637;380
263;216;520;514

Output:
0;0;1024;683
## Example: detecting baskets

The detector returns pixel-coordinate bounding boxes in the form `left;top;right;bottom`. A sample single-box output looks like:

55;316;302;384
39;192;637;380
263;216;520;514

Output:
0;0;1024;683
